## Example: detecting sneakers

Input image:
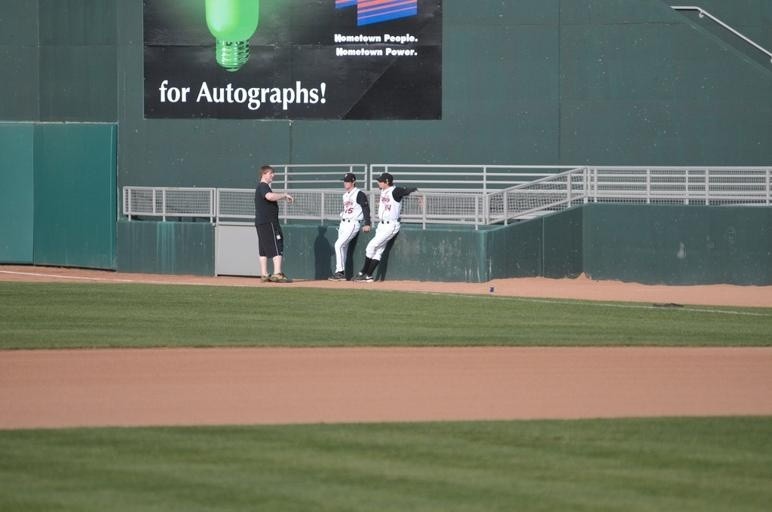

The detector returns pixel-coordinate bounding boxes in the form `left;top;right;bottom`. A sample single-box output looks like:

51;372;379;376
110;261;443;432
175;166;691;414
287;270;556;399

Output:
328;272;347;281
351;271;373;282
261;272;288;283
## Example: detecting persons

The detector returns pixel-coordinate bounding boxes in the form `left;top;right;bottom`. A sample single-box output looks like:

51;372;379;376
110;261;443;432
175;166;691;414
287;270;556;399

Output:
255;165;295;283
327;172;371;282
349;172;424;283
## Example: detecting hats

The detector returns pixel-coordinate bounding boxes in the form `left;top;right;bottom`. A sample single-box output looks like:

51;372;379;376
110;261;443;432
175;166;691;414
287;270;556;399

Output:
376;173;393;183
340;173;356;181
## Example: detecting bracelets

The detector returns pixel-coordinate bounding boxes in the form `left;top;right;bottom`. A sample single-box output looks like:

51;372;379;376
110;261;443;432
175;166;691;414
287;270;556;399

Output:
283;193;287;199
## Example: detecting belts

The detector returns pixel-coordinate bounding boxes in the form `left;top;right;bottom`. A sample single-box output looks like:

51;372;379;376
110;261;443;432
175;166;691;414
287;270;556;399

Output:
382;220;389;224
343;219;350;222
